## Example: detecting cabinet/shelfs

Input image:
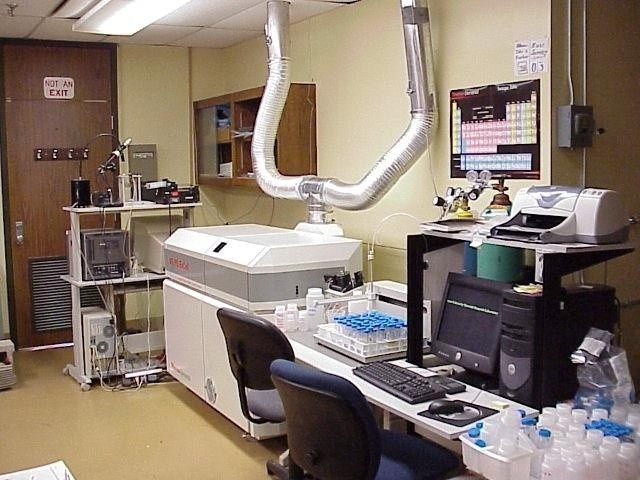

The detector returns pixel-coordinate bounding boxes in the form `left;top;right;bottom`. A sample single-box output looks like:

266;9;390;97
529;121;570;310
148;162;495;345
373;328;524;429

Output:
61;199;204;393
193;82;318;190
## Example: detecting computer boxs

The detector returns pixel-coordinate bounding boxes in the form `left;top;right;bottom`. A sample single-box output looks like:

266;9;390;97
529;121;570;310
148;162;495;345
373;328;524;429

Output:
80;305;119;379
498;283;617;414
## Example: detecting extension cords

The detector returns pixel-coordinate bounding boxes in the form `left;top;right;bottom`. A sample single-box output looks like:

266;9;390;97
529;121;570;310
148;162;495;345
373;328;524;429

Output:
125;368;163;379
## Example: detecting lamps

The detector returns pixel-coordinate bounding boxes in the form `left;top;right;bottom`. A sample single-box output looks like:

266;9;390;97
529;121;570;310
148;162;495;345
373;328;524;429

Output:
72;0;195;39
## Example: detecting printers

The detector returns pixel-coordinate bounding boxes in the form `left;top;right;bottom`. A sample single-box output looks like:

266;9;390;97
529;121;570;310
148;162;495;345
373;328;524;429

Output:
486;184;631;245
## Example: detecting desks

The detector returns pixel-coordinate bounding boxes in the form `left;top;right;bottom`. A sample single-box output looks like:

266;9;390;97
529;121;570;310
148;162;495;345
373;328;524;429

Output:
256;301;541;440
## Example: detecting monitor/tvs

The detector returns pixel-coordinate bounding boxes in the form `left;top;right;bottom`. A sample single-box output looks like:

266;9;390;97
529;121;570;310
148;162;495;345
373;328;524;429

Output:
129;214;183;275
430;271;513;390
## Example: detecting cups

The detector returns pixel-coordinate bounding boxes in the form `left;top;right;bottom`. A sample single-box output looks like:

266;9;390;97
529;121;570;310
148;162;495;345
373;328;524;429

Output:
326;307;346;324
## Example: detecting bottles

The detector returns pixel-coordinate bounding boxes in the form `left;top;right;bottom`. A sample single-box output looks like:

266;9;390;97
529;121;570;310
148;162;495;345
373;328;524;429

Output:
273;287;325;332
348;290;369;315
331;309;409;357
467;394;640;480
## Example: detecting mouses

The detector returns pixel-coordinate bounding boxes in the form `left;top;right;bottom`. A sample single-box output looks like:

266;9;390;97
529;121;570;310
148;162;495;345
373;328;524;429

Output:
428;400;464;414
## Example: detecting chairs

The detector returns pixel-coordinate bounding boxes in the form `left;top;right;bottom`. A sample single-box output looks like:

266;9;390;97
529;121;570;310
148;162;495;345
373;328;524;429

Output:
265;355;461;480
215;307;313;480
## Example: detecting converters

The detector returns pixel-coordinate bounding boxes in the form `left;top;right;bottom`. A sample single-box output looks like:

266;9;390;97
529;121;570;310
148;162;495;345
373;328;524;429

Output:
147;374;158;382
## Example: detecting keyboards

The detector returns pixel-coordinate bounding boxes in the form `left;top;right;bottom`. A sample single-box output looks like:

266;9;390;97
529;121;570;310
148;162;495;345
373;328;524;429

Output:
352;360;446;405
